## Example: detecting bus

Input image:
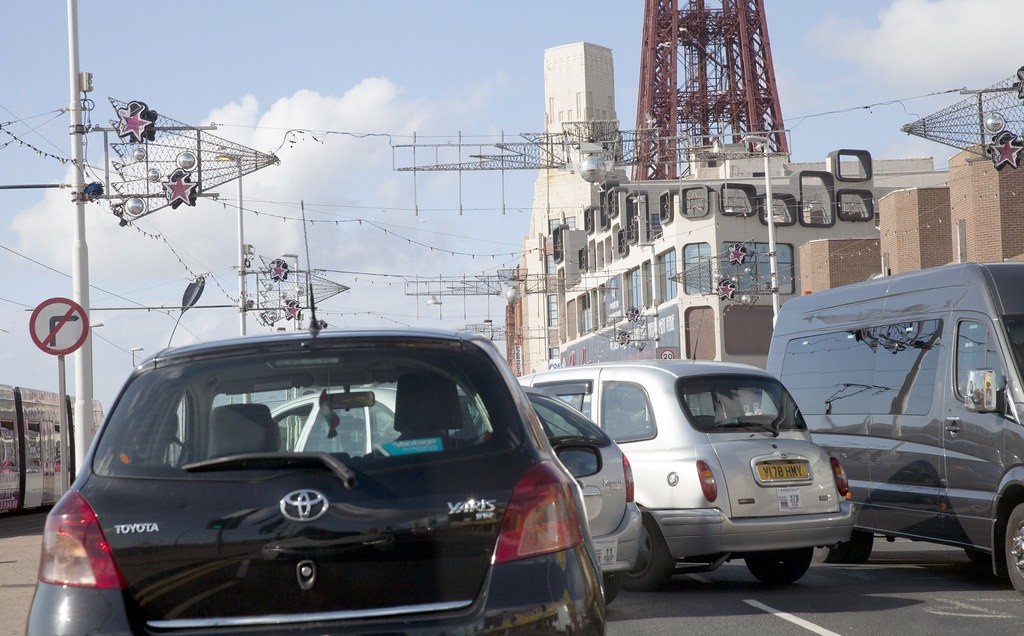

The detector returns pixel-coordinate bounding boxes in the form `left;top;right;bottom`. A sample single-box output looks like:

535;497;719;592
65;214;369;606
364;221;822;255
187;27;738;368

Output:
0;385;107;517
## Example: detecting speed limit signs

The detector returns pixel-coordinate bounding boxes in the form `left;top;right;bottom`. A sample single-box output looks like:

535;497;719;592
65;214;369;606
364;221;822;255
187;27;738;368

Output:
661;349;674;360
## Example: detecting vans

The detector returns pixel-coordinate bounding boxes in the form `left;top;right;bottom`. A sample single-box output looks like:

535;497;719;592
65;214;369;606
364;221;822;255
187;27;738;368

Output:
763;263;1024;594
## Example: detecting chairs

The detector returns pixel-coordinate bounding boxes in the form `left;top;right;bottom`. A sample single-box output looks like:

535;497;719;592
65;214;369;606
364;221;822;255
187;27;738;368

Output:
203;403;281;457
393;371;464;455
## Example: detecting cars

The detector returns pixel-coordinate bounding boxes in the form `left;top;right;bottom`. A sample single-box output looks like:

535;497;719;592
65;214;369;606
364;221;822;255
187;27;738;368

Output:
490;360;856;594
23;325;611;636
383;386;642;608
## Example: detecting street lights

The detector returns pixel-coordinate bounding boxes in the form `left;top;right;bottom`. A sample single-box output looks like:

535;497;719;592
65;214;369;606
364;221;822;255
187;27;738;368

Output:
484;320;494;342
735;133;780;324
281;253;301;335
133;347;145;374
